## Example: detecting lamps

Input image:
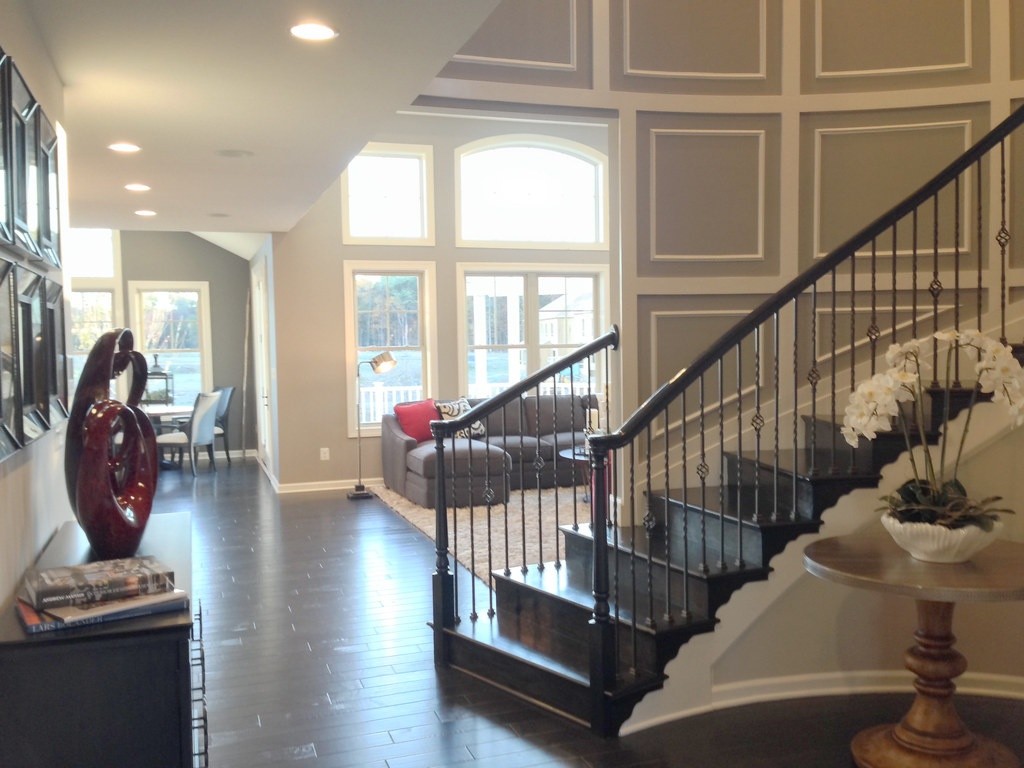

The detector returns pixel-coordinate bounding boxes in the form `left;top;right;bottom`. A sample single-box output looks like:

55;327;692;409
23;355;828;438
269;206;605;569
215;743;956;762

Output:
348;351;398;501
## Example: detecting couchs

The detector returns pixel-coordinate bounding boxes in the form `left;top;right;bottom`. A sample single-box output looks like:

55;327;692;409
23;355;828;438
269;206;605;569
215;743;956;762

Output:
381;394;587;509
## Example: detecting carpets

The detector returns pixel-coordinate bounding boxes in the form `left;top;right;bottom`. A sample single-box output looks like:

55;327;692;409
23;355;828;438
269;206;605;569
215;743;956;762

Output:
367;484;594;593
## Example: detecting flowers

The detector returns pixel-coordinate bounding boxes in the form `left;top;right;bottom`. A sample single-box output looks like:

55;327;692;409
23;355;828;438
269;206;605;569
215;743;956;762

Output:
840;331;1024;522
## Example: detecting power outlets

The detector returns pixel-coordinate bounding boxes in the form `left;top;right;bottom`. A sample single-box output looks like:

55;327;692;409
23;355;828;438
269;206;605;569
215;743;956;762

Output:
320;447;330;460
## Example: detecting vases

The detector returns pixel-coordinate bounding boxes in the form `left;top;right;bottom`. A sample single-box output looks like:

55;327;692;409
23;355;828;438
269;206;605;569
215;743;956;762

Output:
880;507;1003;564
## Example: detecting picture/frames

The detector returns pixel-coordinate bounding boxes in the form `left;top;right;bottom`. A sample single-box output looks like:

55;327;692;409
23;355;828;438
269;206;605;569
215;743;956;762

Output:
0;51;69;464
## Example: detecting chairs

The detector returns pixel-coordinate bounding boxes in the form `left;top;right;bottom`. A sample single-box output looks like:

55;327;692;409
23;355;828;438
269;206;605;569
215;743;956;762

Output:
178;385;235;467
155;389;223;476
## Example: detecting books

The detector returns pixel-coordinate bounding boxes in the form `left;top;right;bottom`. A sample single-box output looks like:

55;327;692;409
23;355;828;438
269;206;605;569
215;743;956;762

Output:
16;556;190;635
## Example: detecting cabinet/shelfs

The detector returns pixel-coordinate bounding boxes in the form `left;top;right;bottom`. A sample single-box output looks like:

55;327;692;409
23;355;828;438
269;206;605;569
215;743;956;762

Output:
0;510;210;768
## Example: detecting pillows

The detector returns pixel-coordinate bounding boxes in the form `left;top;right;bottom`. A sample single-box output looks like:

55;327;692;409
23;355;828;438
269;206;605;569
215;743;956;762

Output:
393;398;442;443
436;395;486;439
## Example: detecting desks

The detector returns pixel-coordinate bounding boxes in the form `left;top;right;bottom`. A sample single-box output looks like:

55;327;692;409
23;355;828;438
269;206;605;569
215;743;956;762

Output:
802;533;1024;768
141;405;194;470
